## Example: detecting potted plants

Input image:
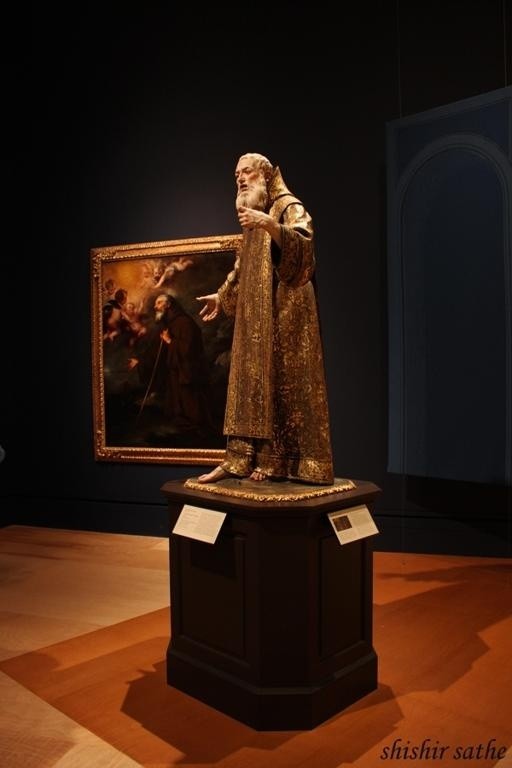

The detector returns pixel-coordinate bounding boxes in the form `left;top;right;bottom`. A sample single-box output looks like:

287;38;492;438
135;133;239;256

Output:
91;233;244;468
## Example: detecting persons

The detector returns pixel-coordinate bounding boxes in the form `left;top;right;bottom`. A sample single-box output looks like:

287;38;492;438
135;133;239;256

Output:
190;152;336;486
96;256;232;449
